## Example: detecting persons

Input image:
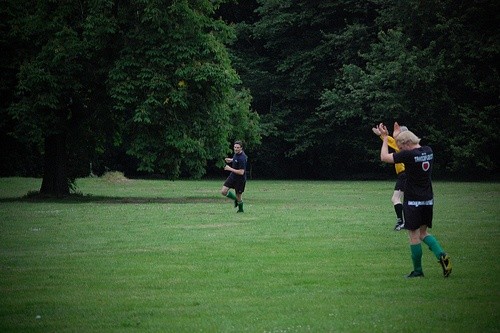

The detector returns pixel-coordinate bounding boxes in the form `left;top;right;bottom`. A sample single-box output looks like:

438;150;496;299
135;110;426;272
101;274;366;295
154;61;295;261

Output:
379;122;452;278
221;140;248;213
372;125;409;229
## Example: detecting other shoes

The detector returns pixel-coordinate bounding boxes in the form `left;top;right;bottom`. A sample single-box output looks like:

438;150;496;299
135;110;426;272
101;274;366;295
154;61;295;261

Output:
235;200;237;207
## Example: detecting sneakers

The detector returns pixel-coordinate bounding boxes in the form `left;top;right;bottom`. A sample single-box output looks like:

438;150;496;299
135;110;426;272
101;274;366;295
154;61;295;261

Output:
394;218;404;230
437;253;452;279
407;271;424;277
